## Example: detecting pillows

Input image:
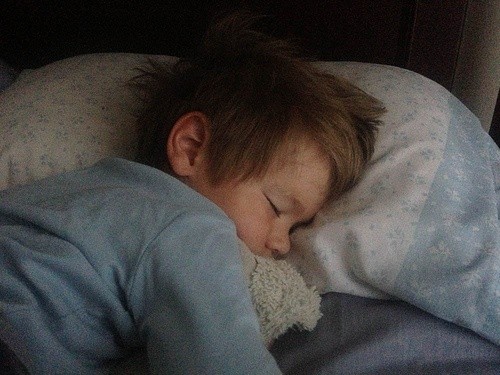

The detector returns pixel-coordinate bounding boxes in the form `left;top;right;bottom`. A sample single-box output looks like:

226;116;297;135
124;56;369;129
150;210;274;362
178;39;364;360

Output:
0;52;499;349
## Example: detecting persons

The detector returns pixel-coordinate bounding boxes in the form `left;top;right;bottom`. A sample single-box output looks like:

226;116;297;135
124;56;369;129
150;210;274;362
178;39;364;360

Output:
1;8;389;375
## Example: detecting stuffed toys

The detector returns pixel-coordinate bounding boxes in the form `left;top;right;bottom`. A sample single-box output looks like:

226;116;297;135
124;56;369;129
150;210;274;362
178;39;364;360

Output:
247;247;323;352
